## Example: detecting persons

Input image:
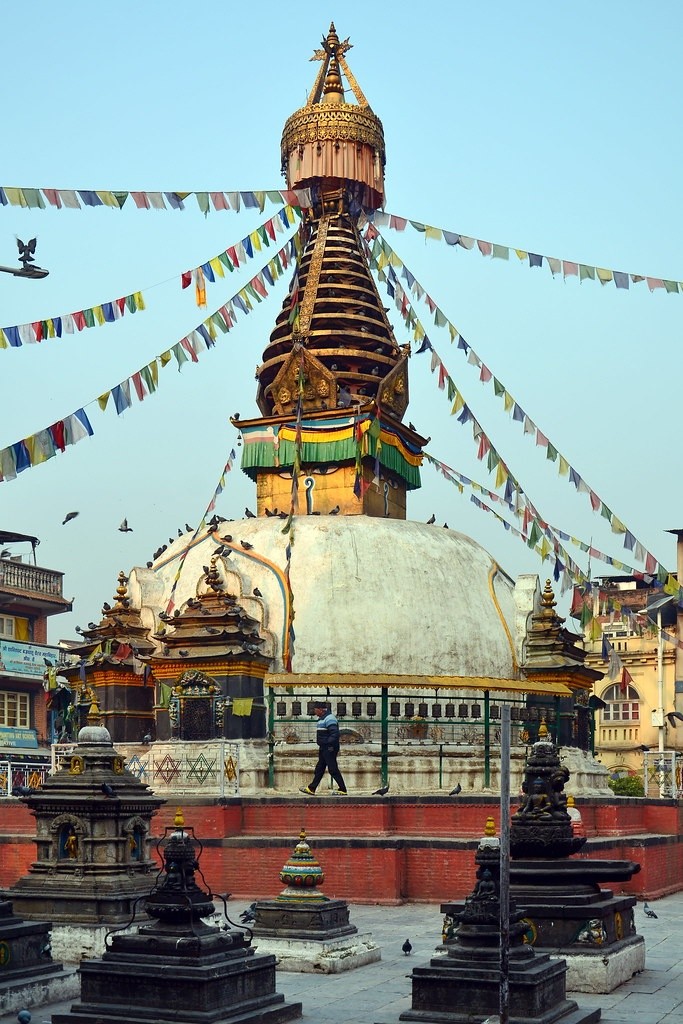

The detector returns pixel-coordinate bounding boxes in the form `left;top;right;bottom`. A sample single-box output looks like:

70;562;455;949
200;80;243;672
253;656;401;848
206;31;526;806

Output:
116;762;121;771
63;829;77;861
510;777;571;820
469;867;498;904
73;762;80;773
298;701;346;795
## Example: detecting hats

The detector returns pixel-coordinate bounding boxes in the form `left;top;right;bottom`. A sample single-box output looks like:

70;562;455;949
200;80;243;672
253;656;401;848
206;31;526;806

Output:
313;701;328;709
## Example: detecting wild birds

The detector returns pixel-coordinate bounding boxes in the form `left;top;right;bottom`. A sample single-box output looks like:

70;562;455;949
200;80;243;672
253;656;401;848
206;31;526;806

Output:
145;522;194;570
371;784;389;796
101;782;119;799
328;505;340;515
639;744;649;752
140;731;152;746
153;565;262;658
206;507;258;558
63;512;79;524
264;507;289;519
17;237;36;262
118;518;133;533
663;712;683;728
443;523;448;528
43;658;53;668
55;576;140;668
644;902;659;919
18;786;34;798
449;783;461;795
383;512;391;518
426;514;436;524
213;893;258;932
401;938;412;955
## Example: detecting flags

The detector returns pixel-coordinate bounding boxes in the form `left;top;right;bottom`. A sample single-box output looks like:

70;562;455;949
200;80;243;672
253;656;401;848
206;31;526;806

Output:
347;196;683;693
232;697;253;717
178;206;304;323
338;390;351;407
276;365;305;675
97;306;234;415
349;401;383;500
0;407;95;484
361;203;683;295
155;449;235;633
0;187;313;217
77;641;173;710
0;291;146;350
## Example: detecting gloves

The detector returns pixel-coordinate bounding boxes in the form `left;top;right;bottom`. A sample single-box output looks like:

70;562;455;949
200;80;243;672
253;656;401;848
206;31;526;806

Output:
327;745;334;754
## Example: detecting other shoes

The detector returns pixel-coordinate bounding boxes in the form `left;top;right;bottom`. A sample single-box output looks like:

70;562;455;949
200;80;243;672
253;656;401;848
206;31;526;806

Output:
298;786;315;796
332;790;347;795
